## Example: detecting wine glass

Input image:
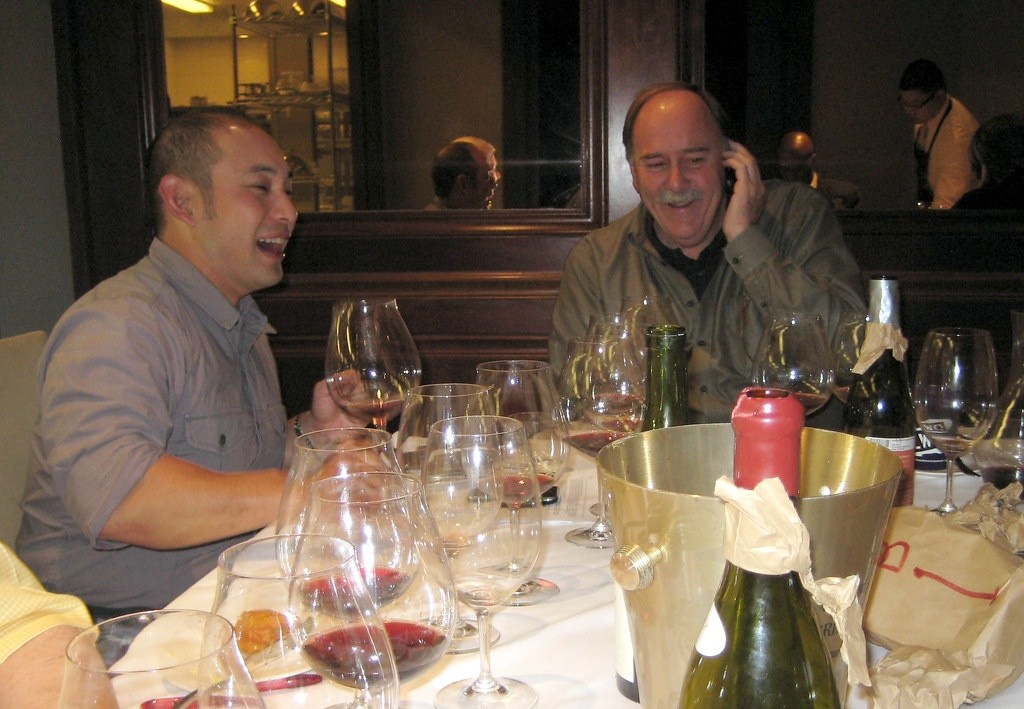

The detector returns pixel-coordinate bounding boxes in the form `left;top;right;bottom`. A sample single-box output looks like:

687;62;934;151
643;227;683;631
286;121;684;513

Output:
913;327;996;516
395;383;503;654
420;415;542;709
324;298;421;430
475;360;570;607
558;296;677;549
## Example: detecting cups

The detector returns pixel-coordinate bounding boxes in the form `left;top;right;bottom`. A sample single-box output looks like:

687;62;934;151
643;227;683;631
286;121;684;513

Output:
275;426;419;617
57;609;266;709
973;409;1024;500
752;312;833;417
286;473;457;688
197;535;399;709
830;308;867;402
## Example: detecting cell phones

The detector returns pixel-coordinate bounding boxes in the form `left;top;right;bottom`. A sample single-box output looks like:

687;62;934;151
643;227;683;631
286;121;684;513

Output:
467;486;557;507
725;166;737;198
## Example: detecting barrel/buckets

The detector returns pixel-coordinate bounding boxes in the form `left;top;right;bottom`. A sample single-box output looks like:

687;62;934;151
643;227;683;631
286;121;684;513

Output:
596;423;902;709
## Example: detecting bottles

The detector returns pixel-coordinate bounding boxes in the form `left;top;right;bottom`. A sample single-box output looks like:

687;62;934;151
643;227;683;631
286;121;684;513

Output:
841;275;915;507
640;324;688;431
678;386;841;709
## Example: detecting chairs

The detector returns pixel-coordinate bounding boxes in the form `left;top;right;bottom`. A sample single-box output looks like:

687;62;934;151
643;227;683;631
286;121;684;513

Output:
0;331;50;554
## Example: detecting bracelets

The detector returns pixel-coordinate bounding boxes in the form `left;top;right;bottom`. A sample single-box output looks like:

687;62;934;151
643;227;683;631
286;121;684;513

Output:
295;412;323;463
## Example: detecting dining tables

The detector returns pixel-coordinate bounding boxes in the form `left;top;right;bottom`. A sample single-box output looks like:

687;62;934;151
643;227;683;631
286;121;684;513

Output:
92;413;1022;709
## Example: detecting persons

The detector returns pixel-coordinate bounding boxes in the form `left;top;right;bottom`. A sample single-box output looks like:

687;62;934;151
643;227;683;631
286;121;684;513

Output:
769;131;859;213
0;541;119;709
15;104;373;671
899;57;1024;210
550;82;867;427
424;137;498;211
